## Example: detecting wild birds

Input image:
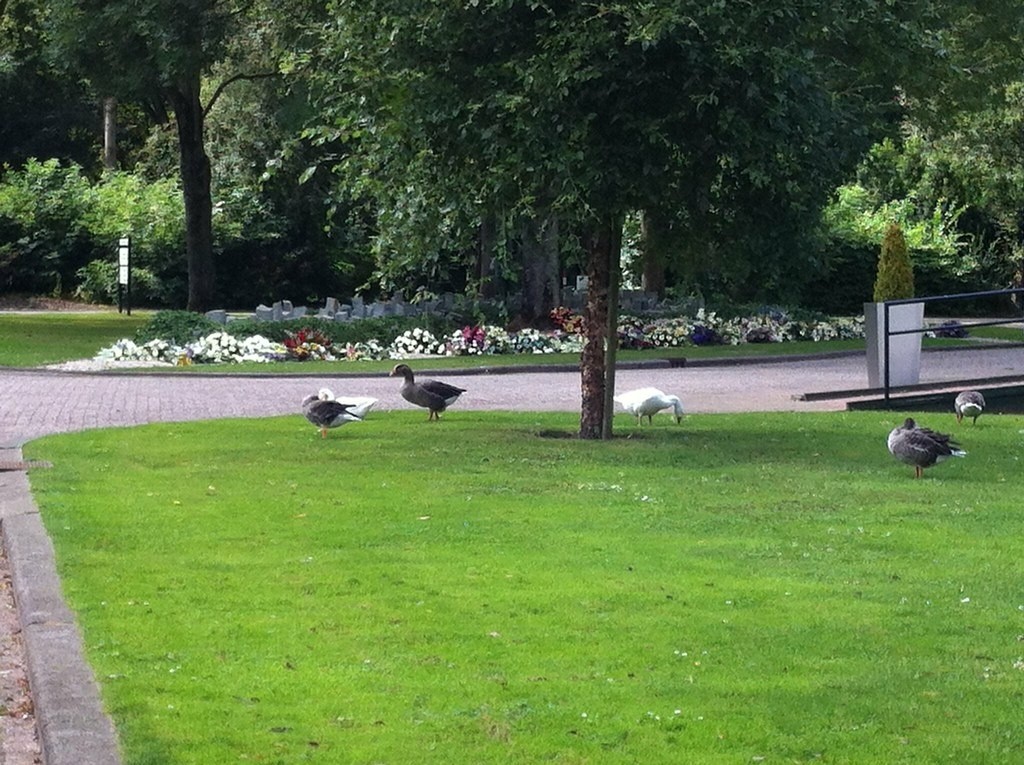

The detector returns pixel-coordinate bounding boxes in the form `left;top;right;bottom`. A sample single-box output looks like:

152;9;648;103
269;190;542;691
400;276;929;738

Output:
388;363;468;422
613;387;685;426
300;388;380;440
885;418;969;475
954;387;989;424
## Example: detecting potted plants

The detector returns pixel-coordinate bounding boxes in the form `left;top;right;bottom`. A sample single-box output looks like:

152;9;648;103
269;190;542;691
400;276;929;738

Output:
863;224;924;388
